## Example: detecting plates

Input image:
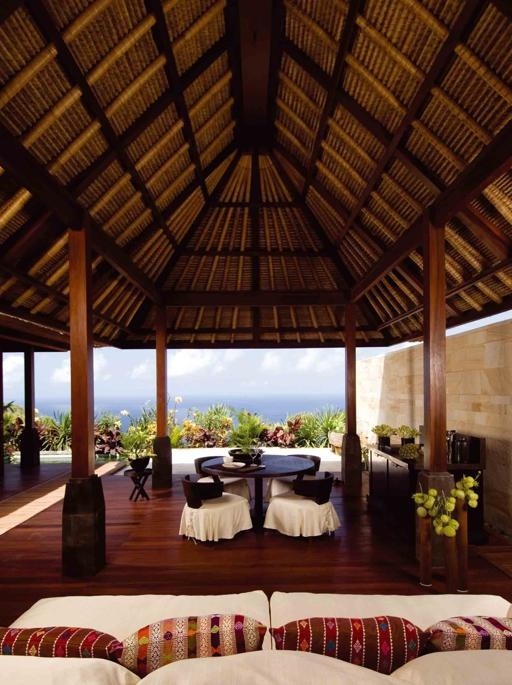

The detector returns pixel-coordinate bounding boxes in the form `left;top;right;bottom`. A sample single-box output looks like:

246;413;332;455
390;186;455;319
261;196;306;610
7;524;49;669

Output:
222;462;246;469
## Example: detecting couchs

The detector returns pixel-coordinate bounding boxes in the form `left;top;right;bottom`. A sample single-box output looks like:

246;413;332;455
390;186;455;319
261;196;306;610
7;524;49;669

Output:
1;584;512;685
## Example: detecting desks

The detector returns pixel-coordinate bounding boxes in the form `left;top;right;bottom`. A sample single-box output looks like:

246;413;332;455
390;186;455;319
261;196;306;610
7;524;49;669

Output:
197;454;316;529
123;468;157;503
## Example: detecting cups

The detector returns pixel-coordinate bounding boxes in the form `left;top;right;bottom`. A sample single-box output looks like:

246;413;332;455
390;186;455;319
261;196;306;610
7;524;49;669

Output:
223;456;234;464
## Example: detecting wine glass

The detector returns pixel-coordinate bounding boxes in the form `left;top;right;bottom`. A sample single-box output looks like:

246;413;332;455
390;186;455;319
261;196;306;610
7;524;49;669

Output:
248;447;258;467
258;441;266;468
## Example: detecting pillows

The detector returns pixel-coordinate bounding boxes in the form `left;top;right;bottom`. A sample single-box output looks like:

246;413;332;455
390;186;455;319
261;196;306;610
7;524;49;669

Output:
118;613;267;679
424;612;512;657
269;614;427;676
1;621;117;660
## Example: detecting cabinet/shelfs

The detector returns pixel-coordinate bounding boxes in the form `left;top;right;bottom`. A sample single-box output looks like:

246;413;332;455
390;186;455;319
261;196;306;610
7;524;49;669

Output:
367;433;486;537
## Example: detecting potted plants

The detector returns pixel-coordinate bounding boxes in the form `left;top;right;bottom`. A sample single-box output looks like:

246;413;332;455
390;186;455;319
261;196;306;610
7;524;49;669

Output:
226;409;268;465
399;443;420;463
114;421;158;469
372;423;396;451
397;423;419;446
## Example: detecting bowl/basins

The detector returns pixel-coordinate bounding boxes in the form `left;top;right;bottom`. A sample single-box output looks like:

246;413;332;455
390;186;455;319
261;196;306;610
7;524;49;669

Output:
228;448;265;459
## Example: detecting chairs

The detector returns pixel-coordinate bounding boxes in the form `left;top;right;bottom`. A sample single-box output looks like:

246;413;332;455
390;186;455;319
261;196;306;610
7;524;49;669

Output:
176;474;253;544
193;455;252;502
258;454;322;500
261;472;341;539
